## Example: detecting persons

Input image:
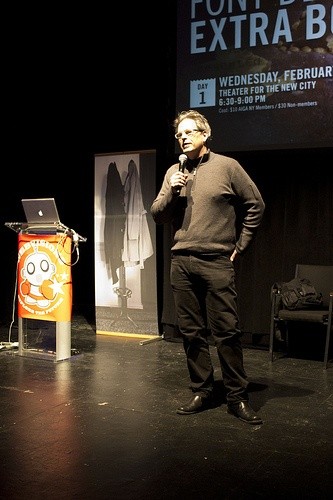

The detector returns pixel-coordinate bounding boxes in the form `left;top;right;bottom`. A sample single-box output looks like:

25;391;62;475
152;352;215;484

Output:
151;110;265;422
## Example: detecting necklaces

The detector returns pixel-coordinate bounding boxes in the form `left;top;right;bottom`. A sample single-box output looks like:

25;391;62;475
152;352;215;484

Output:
189;151;203;176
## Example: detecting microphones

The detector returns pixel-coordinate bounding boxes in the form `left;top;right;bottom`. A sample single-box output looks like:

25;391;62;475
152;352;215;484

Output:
176;154;187;196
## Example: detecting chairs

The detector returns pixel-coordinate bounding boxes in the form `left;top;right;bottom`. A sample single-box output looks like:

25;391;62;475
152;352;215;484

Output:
269;264;333;370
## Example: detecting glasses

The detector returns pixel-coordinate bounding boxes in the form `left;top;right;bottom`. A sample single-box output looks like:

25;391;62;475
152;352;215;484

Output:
174;128;202;139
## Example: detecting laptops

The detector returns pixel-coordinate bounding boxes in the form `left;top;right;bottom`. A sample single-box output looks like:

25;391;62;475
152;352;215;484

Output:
21;198;70;230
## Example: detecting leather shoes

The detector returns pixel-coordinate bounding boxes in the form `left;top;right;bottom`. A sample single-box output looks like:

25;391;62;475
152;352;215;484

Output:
175;395;204;415
227;401;262;423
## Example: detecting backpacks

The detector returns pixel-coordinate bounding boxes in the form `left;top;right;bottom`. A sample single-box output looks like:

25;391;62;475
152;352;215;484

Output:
270;278;322;311
